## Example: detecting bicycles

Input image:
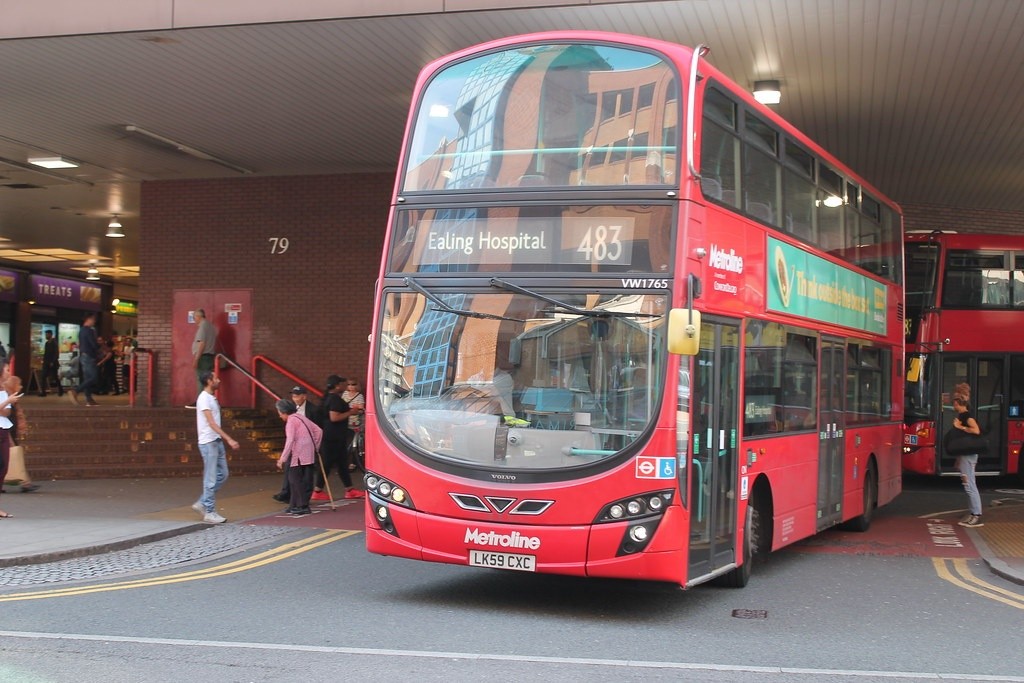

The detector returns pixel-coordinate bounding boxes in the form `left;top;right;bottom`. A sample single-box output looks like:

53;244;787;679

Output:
350;411;365;473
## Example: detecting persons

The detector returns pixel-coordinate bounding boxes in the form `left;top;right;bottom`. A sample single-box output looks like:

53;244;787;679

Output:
341;377;365;471
950;382;984;527
0;358;40;518
38;330;64;397
310;375;365;500
190;369;240;523
96;336;138;395
469;346;516;417
68;312;101;408
275;399;324;514
185;309;216;409
272;385;320;504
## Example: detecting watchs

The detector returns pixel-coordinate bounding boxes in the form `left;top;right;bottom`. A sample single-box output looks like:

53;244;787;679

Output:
962;426;966;431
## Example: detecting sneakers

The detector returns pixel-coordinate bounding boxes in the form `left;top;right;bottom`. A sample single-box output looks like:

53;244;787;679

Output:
957;514;986;528
344;488;366;499
311;490;330;500
192;503;207;517
203;511;227;523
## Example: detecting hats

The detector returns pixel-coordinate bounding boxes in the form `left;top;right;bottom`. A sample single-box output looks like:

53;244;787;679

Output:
289;385;306;395
327;375;347;389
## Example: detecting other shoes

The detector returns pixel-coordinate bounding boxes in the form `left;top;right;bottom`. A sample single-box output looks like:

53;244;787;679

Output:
20;482;40;491
86;401;100;407
68;389;81;408
285;505;312;514
185;403;198;409
271;494;290;504
349;463;356;471
112;392;120;395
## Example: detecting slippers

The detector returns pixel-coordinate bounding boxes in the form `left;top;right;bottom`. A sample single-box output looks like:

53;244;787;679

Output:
0;510;13;518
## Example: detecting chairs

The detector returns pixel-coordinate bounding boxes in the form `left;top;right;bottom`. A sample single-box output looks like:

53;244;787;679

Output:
701;177;858;262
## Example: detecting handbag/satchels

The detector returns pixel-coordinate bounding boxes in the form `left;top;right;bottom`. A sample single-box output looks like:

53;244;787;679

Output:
3;439;27;484
943;417;988;454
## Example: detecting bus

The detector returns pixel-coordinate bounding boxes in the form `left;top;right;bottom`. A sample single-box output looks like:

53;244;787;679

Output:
902;228;1024;490
362;31;910;589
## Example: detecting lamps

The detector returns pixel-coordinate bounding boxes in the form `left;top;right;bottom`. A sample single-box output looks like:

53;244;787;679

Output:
86;264;101;280
105;214;126;238
27;157;80;169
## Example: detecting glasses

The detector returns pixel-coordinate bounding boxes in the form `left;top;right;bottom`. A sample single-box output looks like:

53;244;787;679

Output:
347;382;357;386
